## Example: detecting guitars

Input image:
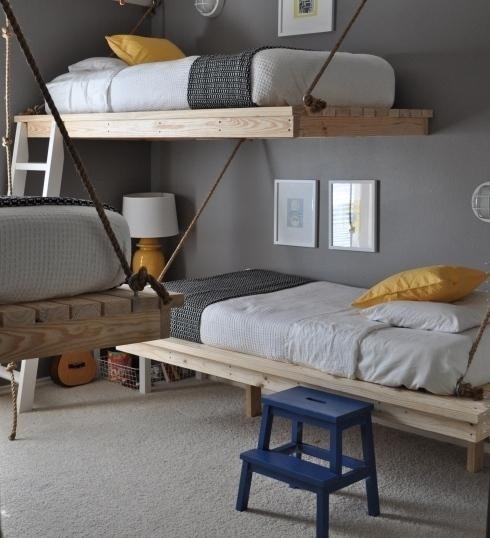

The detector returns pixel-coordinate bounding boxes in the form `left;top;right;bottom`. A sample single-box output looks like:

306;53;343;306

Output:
51;351;96;387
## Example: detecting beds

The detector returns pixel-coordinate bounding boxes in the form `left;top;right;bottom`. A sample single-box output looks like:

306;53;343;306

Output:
114;256;490;474
0;285;184;363
0;103;433;414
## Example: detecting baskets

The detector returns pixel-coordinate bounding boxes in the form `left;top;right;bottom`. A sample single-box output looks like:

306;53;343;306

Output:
92;354;195;389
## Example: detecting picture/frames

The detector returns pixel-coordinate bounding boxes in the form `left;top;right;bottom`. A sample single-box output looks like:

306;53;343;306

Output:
277;0;339;38
328;179;381;255
274;179;319;248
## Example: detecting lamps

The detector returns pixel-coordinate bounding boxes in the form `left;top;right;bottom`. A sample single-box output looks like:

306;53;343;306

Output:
122;192;179;281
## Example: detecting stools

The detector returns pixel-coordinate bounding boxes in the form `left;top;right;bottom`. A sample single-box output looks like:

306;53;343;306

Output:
236;385;380;537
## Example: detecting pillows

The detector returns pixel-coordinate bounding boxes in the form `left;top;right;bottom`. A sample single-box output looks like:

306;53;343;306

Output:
105;34;185;67
361;292;490;332
64;57;124;72
351;264;487;307
